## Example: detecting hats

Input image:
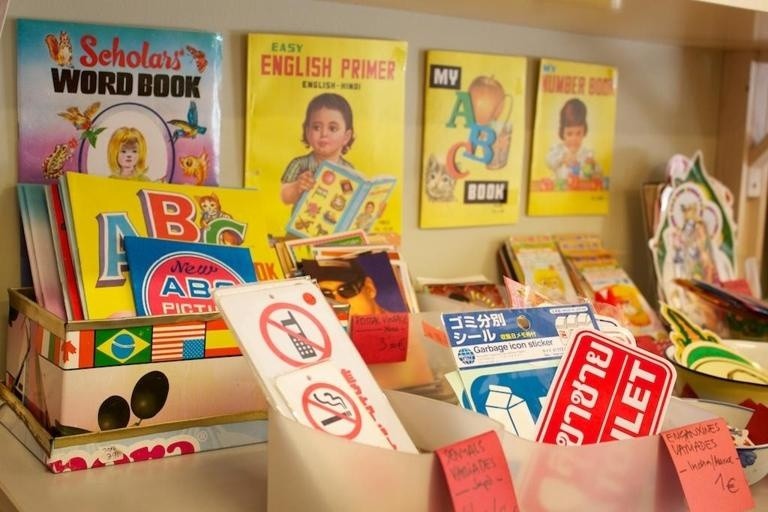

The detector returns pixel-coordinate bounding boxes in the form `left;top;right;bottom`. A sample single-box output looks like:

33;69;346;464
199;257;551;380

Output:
297;258;366;285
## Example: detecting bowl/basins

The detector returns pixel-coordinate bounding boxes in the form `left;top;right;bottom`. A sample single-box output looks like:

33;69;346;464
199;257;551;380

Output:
663;337;767;488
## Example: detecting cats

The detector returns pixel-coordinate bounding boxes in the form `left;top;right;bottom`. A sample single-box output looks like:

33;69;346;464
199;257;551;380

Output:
426;153;460;201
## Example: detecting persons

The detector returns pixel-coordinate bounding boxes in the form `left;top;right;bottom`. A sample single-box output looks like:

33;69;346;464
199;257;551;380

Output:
281;92;359;214
109;125;144;182
550;102;605;188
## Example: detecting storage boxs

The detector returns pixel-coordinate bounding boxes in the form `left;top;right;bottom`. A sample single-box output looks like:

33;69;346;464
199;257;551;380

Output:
2;284;354;476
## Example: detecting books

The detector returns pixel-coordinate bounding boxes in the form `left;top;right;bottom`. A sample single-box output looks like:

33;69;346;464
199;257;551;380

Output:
8;170;768;359
285;158;397;242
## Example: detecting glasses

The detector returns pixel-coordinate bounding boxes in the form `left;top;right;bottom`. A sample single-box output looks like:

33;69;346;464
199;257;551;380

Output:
321;278;365;301
